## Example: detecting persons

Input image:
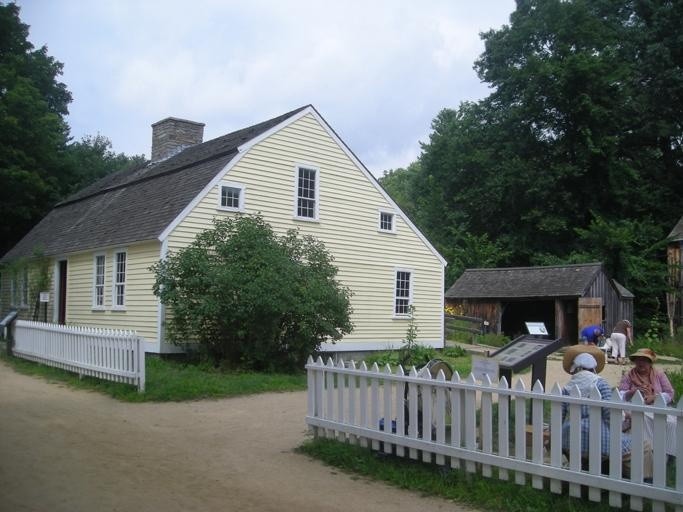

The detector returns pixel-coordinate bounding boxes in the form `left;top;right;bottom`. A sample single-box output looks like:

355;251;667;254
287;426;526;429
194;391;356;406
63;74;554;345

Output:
579;326;604;345
618;348;674;406
551;352;653;480
610;319;633;359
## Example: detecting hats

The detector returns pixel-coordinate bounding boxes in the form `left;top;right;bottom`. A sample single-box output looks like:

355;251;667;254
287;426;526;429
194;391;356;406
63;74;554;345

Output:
627;347;656;364
562;344;605;374
